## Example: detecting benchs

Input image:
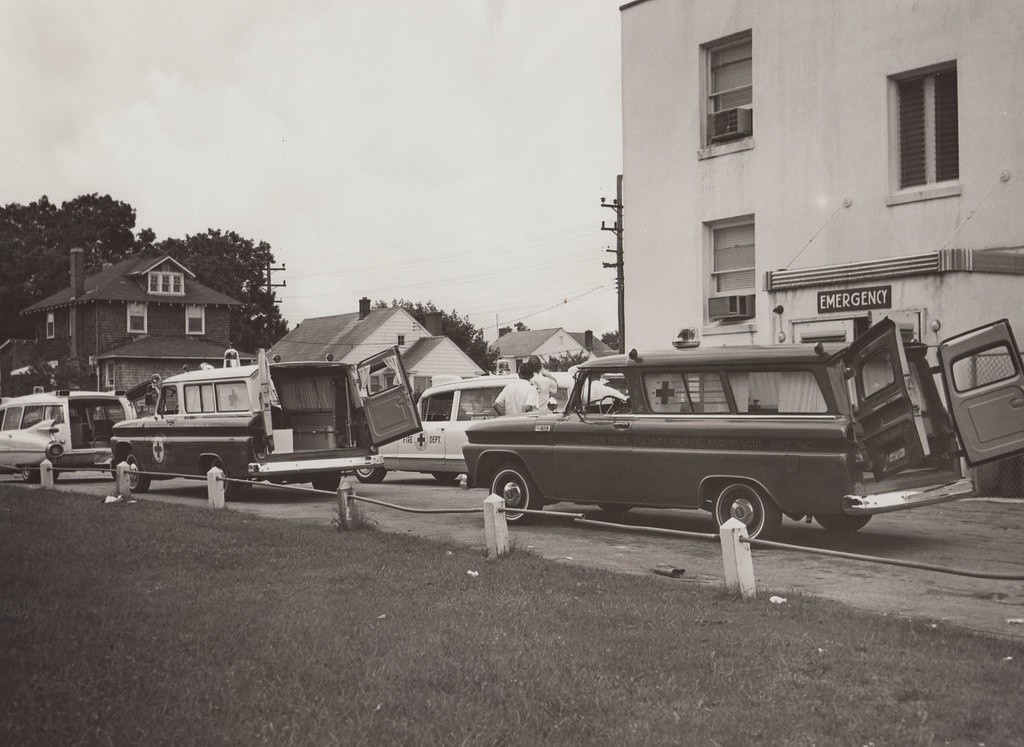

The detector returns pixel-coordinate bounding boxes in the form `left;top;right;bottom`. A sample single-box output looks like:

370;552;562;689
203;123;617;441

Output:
266;425;335;455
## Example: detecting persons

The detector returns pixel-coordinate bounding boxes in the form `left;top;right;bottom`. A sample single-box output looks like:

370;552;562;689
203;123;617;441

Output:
528;355;559;416
580;373;627;406
493;362;539;417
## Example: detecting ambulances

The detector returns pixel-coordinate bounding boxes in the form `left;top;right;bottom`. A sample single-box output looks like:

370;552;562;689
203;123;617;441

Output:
109;348;425;495
351;370;629;484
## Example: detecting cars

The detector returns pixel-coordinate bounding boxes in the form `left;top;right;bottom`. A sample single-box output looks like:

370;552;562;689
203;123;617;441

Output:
0;379;167;484
461;314;1023;548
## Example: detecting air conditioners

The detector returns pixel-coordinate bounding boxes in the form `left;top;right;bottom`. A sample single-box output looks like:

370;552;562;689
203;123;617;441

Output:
708;295;755;319
709;108;752;140
